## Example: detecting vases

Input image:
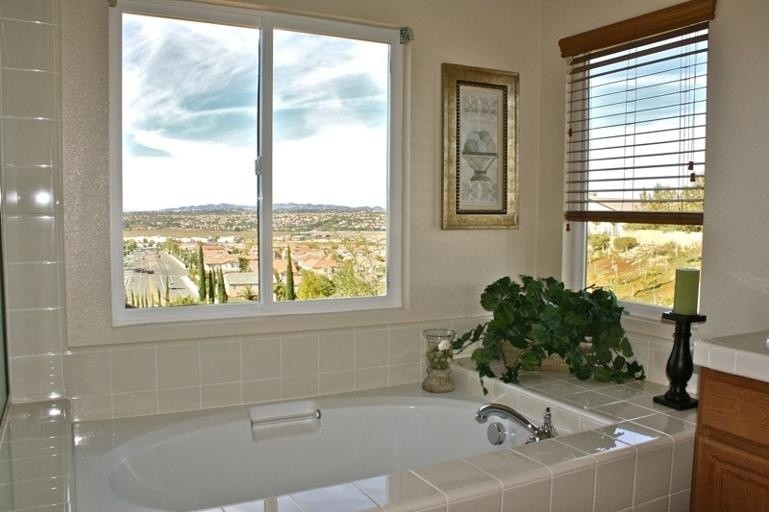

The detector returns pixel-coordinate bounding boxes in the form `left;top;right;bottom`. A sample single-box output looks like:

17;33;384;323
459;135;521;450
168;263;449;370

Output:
422;327;457;393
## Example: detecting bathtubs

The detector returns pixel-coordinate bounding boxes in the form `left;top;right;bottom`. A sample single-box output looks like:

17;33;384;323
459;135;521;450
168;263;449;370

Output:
71;382;575;512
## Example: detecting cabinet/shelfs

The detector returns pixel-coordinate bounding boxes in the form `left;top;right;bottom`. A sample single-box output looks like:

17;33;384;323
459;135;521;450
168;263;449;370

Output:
689;368;769;512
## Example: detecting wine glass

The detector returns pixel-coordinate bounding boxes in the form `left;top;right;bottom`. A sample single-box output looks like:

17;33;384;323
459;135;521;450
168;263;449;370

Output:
460;151;497;182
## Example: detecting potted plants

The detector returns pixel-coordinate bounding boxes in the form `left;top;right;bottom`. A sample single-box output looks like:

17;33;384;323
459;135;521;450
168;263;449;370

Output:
449;272;647;395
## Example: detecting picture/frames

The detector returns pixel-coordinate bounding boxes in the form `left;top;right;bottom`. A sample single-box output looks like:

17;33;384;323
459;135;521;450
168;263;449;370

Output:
440;62;521;230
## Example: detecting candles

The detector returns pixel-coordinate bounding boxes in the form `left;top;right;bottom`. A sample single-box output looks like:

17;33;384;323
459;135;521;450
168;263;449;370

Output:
672;267;699;315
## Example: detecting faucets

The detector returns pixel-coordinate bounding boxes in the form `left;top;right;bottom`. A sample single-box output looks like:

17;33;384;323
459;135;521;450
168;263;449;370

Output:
474;401;559;444
